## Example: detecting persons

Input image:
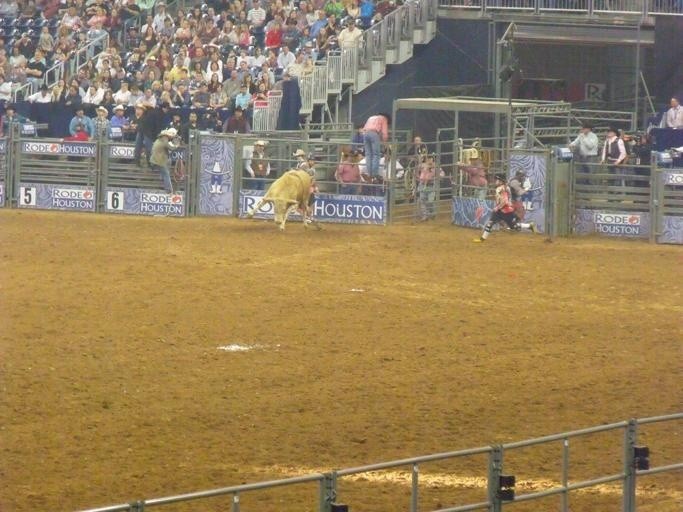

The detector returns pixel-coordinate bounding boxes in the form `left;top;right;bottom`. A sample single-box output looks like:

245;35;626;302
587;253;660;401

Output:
240;97;681;244
2;1;407;192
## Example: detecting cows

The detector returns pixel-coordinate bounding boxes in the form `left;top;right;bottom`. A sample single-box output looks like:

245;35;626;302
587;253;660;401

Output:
246;169;321;234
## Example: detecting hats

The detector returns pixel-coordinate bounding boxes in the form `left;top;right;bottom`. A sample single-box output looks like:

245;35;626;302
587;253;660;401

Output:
608;128;621;137
147;55;159;62
582;122;595;129
253;140;266;147
156;2;167;8
112;104;127;113
234;105;245;112
4;105;16;113
292;148;306;157
494;173;509;184
94;105;109;116
378;111;393;124
345;150;358;157
37;84;50;91
158;127;178;138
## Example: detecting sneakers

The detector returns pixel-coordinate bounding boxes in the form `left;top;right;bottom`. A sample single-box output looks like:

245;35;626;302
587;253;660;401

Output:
472;238;485;244
529;222;537;234
